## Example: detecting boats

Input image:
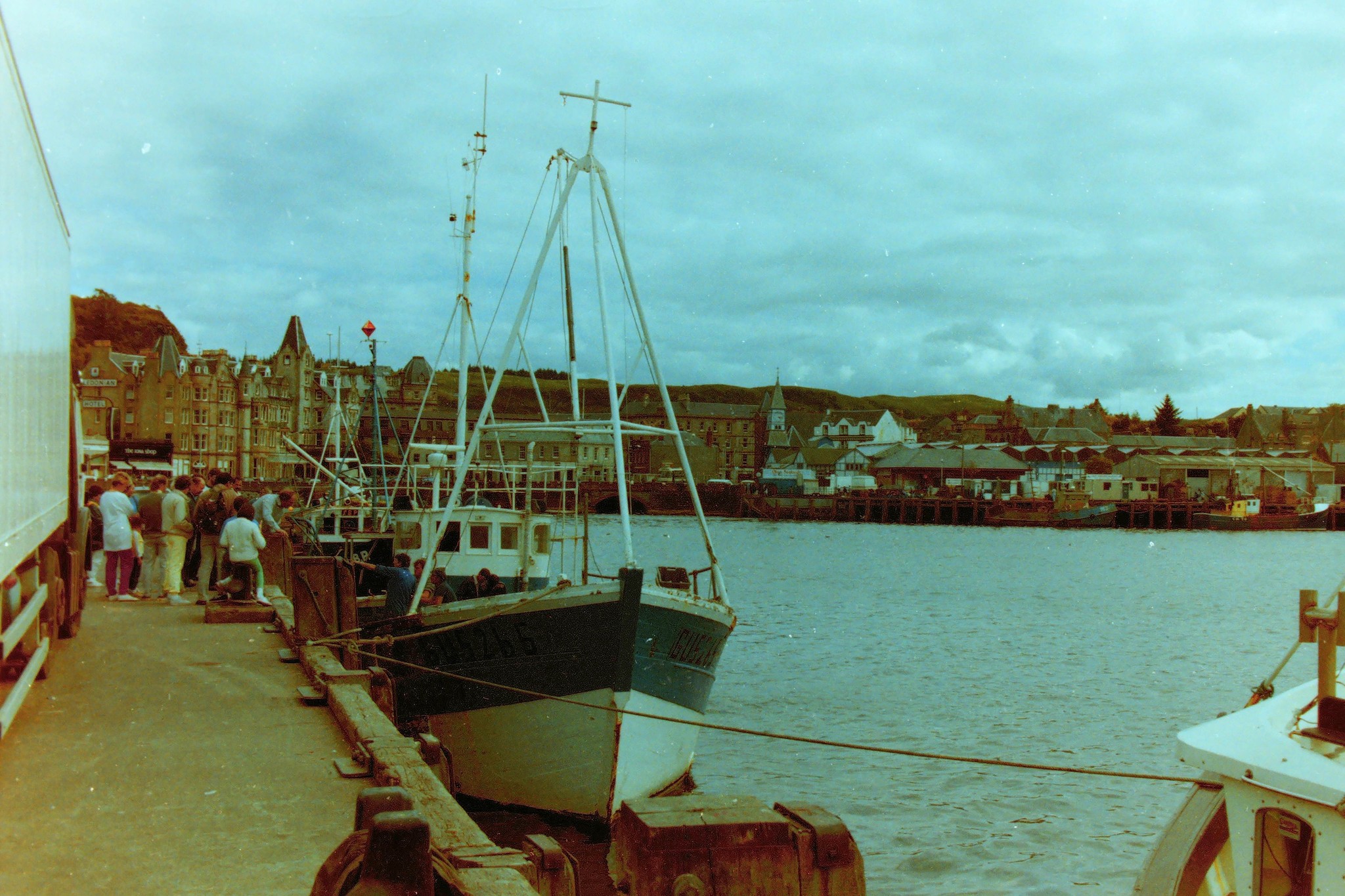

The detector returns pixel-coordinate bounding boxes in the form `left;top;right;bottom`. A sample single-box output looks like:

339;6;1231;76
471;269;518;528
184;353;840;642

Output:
1190;448;1330;532
984;453;1118;529
1133;576;1345;896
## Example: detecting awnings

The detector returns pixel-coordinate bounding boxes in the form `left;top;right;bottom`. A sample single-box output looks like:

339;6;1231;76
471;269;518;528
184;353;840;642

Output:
109;461;132;469
129;461;173;471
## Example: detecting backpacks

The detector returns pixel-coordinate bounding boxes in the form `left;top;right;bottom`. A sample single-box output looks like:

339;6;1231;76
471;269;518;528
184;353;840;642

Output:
196;489;228;534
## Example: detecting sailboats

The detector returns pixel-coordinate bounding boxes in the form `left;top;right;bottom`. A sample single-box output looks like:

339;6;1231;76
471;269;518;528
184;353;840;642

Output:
287;67;737;833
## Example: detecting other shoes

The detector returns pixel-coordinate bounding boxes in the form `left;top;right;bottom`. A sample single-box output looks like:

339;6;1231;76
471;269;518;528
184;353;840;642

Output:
140;595;151;601
196;599;207;605
256;596;274;606
157;595;167;601
117;594;139;601
167;593;192;604
215;580;225;595
128;588;144;599
108;594;119;601
86;582;102;587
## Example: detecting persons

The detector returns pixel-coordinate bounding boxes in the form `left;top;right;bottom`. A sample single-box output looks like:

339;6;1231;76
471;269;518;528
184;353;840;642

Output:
83;467;296;607
353;553;507;618
